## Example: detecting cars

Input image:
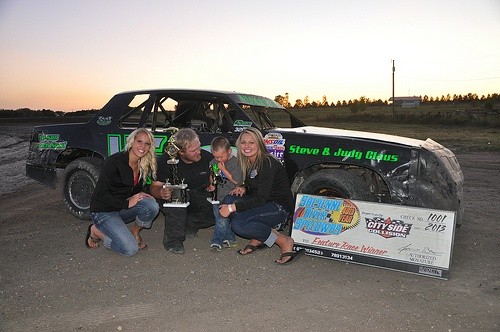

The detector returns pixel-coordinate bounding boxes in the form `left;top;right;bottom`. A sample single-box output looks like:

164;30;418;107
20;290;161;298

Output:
24;86;464;229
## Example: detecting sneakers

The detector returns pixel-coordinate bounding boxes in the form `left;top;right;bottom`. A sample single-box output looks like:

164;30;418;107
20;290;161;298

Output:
209;244;222;252
223;240;237;247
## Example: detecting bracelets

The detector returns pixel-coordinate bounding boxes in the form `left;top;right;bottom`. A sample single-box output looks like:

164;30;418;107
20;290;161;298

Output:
228;204;233;212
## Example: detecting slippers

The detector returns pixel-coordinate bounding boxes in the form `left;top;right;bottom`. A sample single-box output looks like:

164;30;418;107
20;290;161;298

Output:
134;234;147;250
85;223;101;249
237;243;267;255
275;249;306;265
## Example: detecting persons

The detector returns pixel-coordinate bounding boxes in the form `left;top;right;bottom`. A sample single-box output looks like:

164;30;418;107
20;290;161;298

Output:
85;128;164;256
209;136;243;252
150;128;216;254
218;128;306;265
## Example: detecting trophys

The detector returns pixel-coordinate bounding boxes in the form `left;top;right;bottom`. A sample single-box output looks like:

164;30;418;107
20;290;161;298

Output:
163;137;189;207
207;164;221;204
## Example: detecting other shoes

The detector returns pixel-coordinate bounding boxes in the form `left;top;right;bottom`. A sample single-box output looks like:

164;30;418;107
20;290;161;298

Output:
168;244;185;254
186;231;199;239
